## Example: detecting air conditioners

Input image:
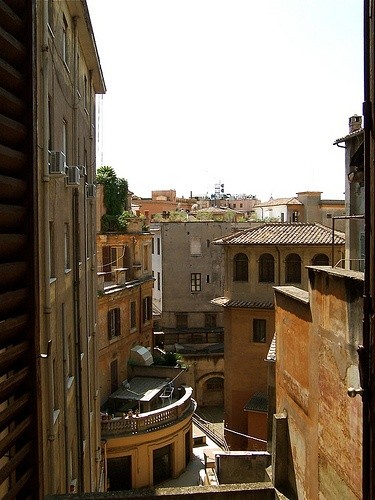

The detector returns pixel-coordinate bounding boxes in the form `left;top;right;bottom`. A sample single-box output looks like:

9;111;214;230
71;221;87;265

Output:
86;184;96;198
79;165;86;176
49;150;66;174
66;166;80;186
